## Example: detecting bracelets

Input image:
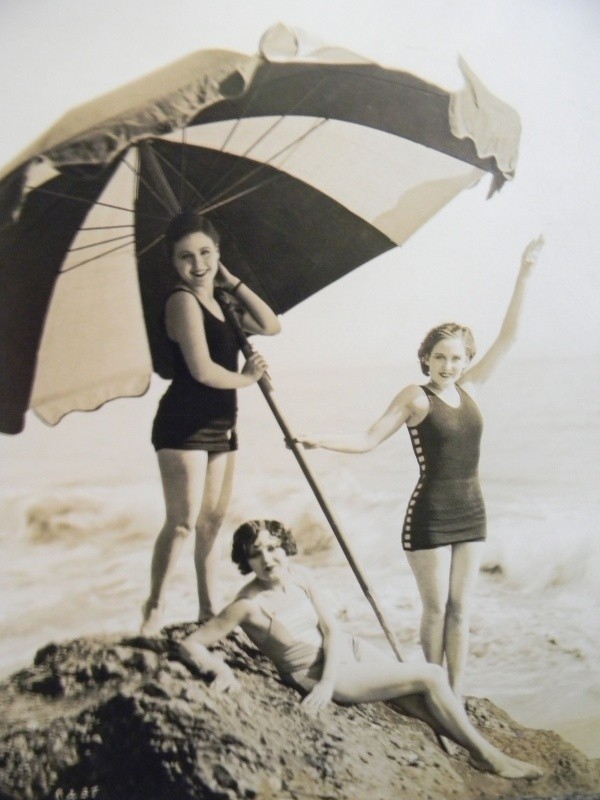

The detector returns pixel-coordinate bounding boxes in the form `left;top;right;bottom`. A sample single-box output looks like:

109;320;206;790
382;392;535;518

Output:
230;278;244;293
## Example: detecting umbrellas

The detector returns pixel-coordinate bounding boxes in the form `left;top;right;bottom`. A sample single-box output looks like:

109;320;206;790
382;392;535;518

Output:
0;23;532;670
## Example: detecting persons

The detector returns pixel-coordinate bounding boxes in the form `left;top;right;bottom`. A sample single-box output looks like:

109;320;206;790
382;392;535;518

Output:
283;233;550;702
140;212;280;642
177;521;548;779
